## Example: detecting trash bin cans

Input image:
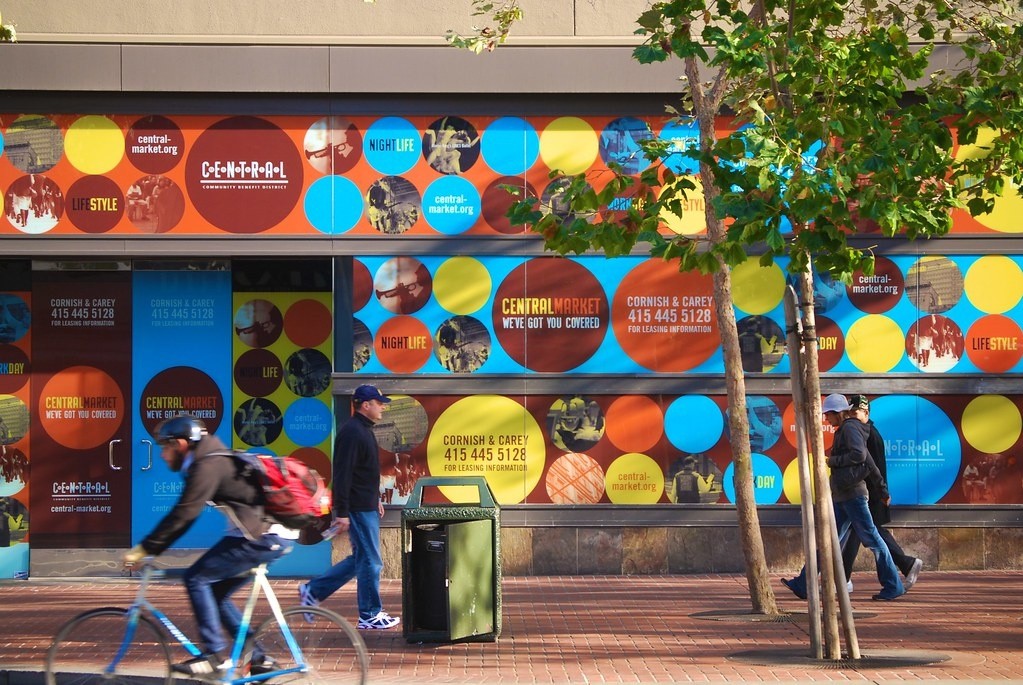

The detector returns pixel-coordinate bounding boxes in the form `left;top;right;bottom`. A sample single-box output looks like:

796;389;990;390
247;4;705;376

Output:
402;475;502;644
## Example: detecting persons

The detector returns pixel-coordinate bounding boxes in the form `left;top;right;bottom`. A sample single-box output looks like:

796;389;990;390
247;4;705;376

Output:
440;318;488;374
906;314;964;367
237;398;282;445
572;400;604;431
746;396;783;450
738;319;776;373
124;416;300;685
298;384;400;628
369;177;417;233
0;444;29;485
289;357;315;396
781;393;922;600
671;457;713;503
7;173;62;227
380;452;425;504
0;499;23;548
126;175;169;221
599;119;651;173
353;345;370;372
963;454;1006;501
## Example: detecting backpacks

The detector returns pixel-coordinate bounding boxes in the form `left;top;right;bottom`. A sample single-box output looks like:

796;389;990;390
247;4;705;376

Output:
194;448;332;545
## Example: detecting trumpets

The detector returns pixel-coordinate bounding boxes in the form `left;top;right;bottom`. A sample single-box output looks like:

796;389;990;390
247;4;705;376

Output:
235;319;272;336
375;281;419;300
305;141;348;160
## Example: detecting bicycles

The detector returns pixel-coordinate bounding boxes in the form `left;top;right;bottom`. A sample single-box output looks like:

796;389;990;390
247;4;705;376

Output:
44;545;370;685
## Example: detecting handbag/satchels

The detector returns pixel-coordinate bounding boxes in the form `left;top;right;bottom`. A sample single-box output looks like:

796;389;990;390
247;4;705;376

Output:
831;426;874;491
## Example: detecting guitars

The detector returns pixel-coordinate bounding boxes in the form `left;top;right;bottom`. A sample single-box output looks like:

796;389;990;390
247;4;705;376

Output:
367;200;403;222
438;341;473;362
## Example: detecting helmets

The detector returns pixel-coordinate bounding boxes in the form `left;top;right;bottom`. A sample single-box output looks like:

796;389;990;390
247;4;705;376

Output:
154;416;208;444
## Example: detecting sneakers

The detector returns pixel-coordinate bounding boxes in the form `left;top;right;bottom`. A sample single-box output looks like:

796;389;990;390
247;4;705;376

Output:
173;652;232;678
355;613;401;629
298;583;321;623
245;653;276;685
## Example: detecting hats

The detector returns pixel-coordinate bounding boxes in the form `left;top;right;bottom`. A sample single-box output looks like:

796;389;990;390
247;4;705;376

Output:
848;395;870;412
354;385;391;403
822;394;851;414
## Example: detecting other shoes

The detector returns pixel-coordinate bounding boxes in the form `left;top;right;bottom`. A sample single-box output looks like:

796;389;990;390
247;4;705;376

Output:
904;558;924;590
872;593;889;603
780;578;808;599
819;578;854;595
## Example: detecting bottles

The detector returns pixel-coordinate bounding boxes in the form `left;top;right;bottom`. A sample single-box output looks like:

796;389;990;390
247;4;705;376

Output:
322;525;340;541
